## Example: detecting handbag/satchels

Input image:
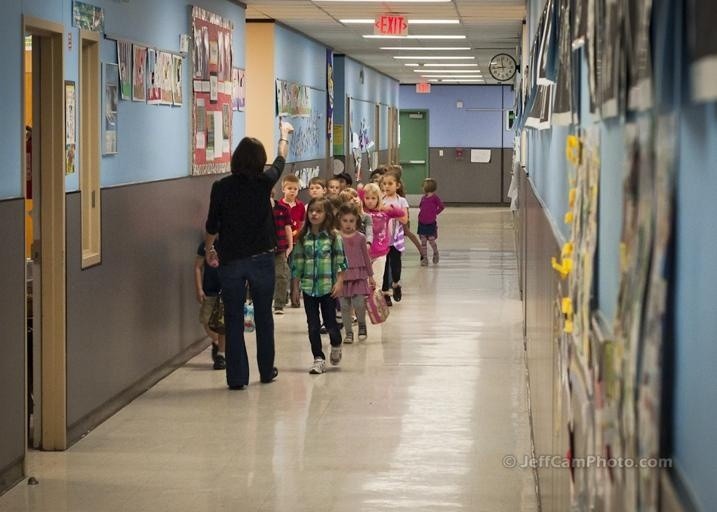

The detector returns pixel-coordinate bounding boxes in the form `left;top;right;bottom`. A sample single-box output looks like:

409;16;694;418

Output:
365;288;389;323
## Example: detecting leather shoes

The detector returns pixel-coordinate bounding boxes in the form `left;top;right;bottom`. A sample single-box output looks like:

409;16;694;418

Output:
261;368;278;383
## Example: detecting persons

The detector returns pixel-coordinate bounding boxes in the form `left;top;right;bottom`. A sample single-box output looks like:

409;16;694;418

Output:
202;115;296;392
188;163;447;373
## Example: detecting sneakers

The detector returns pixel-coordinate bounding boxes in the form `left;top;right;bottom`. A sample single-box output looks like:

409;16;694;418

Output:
433;255;439;264
310;318;367;374
421;257;428;266
274;307;284;314
212;342;227;369
391;281;401;301
384;295;392;306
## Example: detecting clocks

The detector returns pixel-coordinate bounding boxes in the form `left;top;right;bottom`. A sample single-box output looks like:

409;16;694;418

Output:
488;53;519;83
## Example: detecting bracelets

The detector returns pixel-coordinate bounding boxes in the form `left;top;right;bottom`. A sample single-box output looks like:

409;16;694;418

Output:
203;245;215;251
278;138;289;145
